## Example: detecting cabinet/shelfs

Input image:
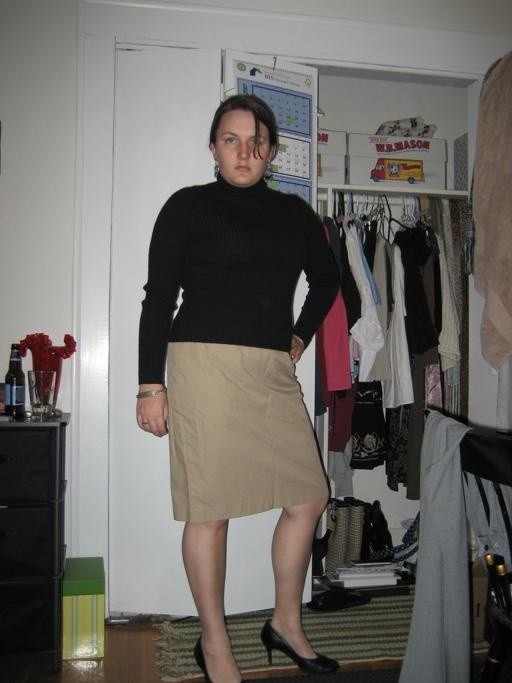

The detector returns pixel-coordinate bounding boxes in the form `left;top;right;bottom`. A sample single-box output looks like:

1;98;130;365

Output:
74;1;498;622
0;412;68;683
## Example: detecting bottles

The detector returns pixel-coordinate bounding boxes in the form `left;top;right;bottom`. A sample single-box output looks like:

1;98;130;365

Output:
5;343;27;423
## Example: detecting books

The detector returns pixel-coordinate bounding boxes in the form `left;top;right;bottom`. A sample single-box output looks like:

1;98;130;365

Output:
329;557;406;586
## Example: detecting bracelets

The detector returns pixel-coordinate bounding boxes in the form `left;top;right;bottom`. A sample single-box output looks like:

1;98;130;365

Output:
136;390;164;398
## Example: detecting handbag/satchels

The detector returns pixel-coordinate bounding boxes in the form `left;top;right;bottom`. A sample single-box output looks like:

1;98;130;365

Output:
361;500;395;563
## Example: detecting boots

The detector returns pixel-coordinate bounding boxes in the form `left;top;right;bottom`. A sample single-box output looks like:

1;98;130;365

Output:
344;496;366;565
327;500;350;575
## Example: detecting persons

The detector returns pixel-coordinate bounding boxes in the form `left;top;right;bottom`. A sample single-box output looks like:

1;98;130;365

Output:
134;92;341;681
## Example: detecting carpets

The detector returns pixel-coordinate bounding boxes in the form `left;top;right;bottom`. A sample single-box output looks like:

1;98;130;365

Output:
154;587;489;683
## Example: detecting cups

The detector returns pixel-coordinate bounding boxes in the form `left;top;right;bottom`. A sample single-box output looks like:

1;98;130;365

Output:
28;370;57;422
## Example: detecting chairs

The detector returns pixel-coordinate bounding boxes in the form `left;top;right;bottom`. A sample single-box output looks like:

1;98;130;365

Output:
421;408;512;682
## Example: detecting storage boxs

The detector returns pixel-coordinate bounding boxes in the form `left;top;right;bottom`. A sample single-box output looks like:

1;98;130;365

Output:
312;131;347;183
60;558;105;660
347;134;448;190
472;577;489;641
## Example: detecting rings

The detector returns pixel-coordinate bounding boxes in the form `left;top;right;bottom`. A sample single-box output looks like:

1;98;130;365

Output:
291;355;295;359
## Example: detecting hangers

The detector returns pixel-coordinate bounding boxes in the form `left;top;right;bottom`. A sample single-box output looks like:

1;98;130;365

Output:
316;191;433;242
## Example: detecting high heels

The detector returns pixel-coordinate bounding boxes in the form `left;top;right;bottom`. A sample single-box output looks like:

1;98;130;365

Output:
193;633;243;683
260;617;340;674
